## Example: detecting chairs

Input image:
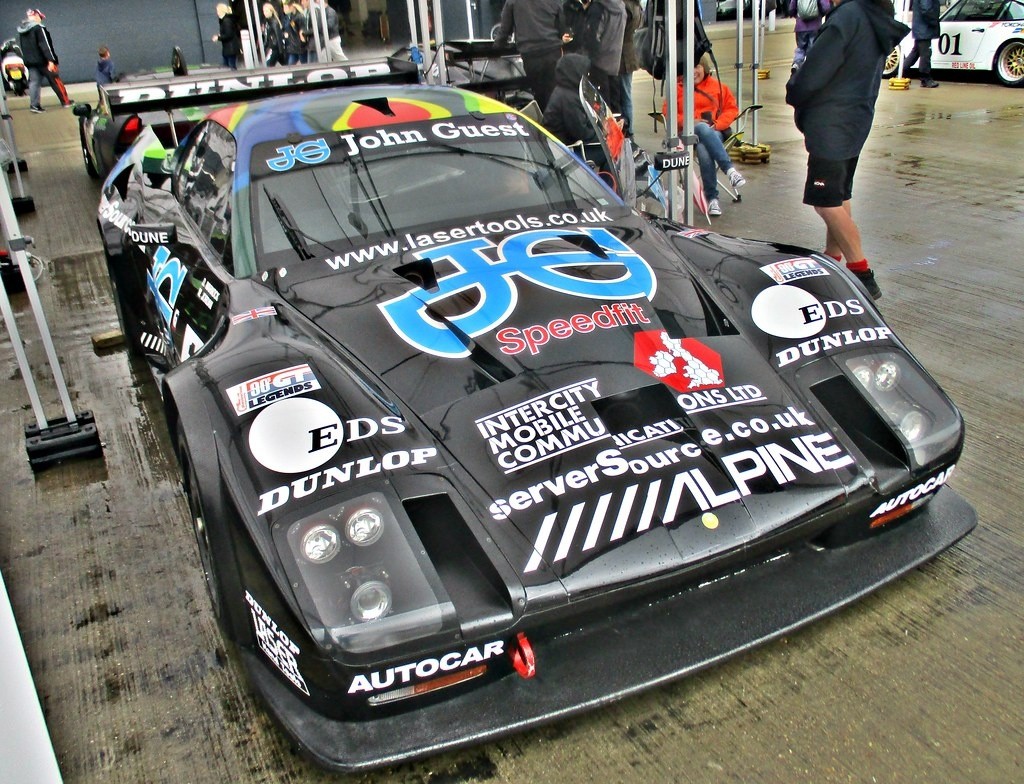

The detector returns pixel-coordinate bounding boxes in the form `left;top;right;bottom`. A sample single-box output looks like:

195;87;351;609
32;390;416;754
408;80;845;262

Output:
519;101;624;176
648;105;763;203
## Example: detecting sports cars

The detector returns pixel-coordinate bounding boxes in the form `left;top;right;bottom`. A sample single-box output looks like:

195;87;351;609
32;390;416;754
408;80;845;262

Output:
93;57;981;775
881;0;1024;88
72;100;191;183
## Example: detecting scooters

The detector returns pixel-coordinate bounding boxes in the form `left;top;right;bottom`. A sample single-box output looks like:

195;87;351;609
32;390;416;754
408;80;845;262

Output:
0;38;29;98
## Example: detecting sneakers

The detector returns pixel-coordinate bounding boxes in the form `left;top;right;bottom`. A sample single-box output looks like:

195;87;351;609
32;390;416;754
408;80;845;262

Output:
30;104;47;114
707;199;722;215
62;100;76;108
853;269;882;300
728;171;746;188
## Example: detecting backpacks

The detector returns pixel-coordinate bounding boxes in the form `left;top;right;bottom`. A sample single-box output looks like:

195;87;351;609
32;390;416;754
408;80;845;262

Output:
797;0;818;21
632;0;722;133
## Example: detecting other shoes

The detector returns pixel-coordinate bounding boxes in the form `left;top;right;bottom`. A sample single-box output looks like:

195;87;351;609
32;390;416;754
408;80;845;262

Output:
625;133;634;143
902;76;911;83
791;64;799;73
920;79;939;88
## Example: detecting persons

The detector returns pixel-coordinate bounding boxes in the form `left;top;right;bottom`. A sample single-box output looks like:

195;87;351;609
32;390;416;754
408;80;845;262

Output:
17;7;75;113
261;0;348;66
786;0;911;300
95;45;120;110
788;0;831;78
540;54;624;200
492;0;643;141
213;3;239;69
902;0;940;88
662;56;746;215
